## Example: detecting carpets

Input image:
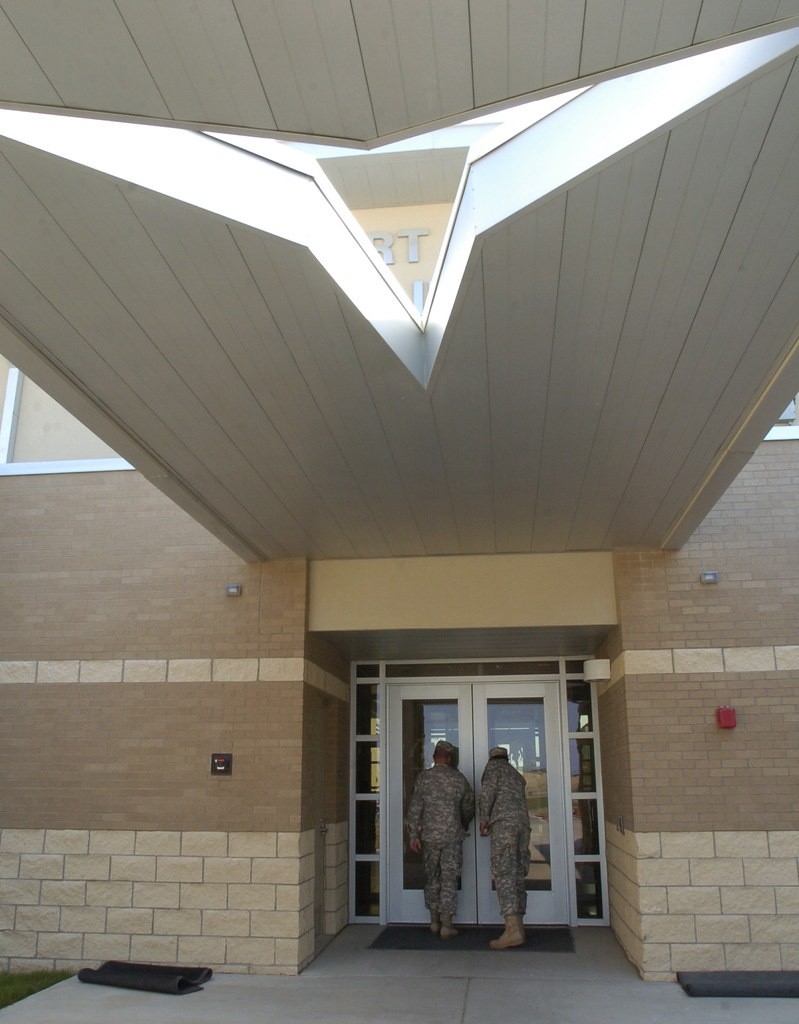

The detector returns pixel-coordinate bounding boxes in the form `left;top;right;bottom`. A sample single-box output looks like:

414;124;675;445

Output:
367;926;576;953
78;961;214;995
676;970;799;998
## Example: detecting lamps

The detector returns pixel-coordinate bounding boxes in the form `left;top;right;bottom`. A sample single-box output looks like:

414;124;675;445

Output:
700;571;719;584
226;583;242;597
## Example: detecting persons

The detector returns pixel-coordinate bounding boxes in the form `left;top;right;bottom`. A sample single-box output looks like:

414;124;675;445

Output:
477;747;532;949
407;739;475;937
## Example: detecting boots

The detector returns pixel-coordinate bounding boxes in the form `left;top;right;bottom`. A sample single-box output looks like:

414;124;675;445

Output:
490;913;525;949
430;909;458;940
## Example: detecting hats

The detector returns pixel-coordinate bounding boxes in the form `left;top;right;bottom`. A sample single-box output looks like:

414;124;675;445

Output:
489;747;507;757
435;741;452;752
